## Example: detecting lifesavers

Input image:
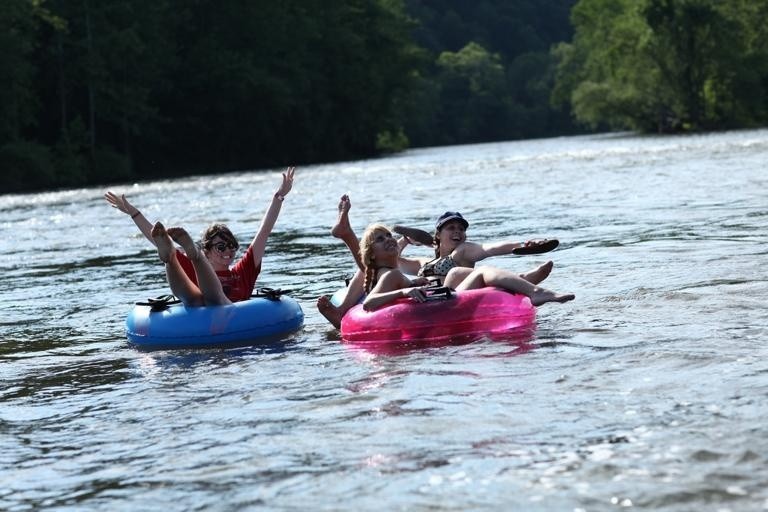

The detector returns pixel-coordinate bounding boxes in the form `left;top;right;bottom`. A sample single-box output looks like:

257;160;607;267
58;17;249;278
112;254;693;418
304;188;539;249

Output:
330;259;448;309
341;286;535;345
124;289;304;349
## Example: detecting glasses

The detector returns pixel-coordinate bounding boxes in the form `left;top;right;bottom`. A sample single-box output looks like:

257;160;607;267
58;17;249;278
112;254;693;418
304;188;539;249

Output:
209;241;237;252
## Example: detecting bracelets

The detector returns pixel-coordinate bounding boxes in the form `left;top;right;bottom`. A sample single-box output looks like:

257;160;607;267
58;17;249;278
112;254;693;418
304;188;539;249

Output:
132;211;140;219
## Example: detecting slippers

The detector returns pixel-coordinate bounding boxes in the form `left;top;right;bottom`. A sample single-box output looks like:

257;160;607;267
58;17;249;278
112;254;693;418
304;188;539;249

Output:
393;225;433;247
136;295;182;306
252;287;293;296
512;238;560;255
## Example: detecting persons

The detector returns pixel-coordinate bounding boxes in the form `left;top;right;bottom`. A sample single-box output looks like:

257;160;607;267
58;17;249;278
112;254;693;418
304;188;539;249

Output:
359;225;574;311
317;195;559;329
105;165;295;306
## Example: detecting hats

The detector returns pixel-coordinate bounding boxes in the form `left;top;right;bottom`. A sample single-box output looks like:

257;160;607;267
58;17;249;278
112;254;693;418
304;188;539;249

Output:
435;212;469;231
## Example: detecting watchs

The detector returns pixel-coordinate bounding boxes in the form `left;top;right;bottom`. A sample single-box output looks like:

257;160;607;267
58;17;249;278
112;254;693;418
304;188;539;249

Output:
275;193;284;201
